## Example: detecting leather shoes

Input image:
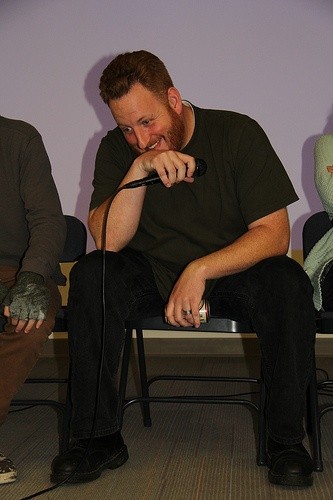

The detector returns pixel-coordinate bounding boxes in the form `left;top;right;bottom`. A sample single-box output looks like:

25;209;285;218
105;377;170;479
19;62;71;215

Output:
266;442;313;486
49;430;129;482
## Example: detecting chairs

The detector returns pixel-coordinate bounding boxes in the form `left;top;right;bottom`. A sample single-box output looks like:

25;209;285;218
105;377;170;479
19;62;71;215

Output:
10;211;333;471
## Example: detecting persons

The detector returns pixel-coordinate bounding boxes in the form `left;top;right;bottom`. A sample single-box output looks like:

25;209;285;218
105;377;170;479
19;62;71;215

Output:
301;133;333;321
0;115;68;486
48;50;318;489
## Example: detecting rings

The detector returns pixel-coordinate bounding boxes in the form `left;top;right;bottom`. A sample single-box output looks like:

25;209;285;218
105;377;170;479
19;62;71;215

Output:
183;310;192;315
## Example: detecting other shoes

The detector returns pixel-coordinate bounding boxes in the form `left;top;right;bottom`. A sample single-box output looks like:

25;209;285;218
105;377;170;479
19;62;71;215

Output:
0;451;17;483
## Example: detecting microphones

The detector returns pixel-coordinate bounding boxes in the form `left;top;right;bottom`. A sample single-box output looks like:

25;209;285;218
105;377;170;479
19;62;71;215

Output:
124;158;207;188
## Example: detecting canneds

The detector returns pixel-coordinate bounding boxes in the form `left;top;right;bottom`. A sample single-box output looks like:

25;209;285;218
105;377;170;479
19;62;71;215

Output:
165;298;210;324
